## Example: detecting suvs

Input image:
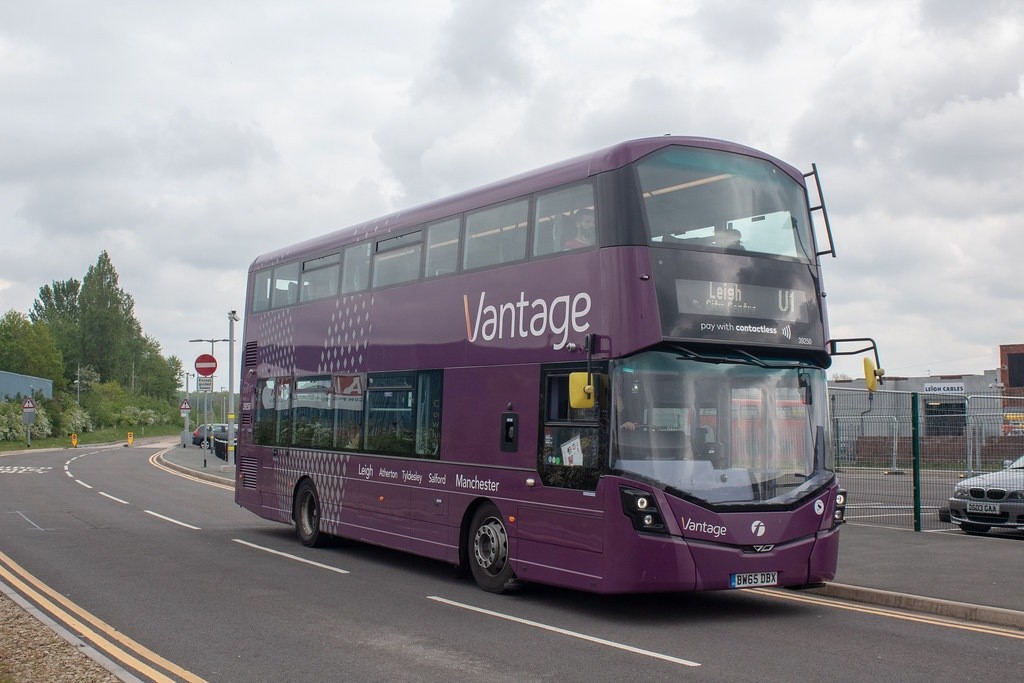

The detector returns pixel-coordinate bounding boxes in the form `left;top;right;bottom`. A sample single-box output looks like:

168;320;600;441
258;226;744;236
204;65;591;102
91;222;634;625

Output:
191;424;241;450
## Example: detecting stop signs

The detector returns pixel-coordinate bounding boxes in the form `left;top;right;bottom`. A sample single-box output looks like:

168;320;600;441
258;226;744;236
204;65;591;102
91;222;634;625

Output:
195;354;220;378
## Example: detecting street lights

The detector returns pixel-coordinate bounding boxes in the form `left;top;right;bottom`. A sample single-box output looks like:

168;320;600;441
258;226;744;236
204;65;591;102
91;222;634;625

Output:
220;386;228;423
189;339;241;468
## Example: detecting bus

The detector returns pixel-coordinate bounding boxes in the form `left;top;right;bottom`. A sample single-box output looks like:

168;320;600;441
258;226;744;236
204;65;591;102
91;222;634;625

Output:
234;136;885;598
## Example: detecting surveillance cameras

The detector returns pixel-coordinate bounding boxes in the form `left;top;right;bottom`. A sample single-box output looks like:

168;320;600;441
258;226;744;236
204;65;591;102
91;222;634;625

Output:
989;384;992;388
233;315;240;321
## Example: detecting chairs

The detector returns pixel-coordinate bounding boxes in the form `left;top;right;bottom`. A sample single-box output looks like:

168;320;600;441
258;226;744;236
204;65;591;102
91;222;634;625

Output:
270;213;574;309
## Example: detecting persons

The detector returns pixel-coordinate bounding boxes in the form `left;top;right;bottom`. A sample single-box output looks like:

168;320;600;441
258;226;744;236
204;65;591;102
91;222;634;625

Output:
614;396;638;433
562;208;594;252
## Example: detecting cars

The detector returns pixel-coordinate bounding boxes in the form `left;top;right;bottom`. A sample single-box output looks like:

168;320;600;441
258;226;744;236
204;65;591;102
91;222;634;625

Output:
948;456;1024;532
